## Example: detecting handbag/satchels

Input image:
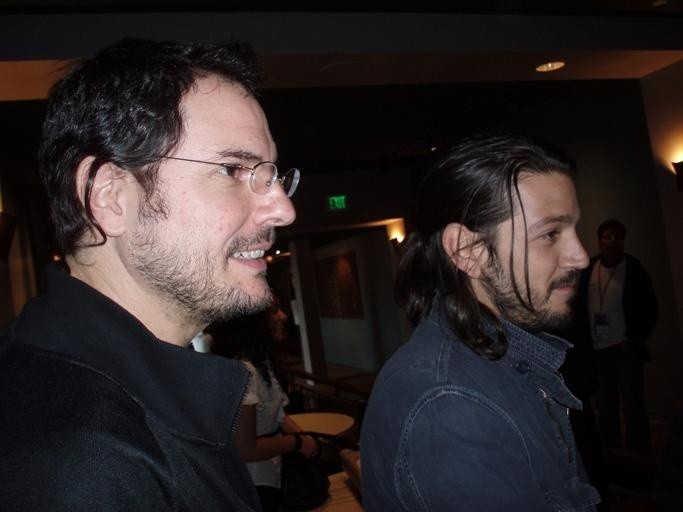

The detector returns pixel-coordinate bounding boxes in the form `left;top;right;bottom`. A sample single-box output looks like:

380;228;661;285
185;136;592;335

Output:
284;451;330;510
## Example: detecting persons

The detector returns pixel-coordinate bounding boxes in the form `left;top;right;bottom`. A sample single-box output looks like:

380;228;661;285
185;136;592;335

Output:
0;36;300;512
208;273;323;506
359;124;606;511
573;218;660;461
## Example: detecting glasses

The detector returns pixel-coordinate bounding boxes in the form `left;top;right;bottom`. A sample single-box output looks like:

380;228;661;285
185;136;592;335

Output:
159;156;301;197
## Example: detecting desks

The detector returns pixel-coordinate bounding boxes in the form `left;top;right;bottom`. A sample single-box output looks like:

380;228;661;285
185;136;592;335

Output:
285;413;356;474
